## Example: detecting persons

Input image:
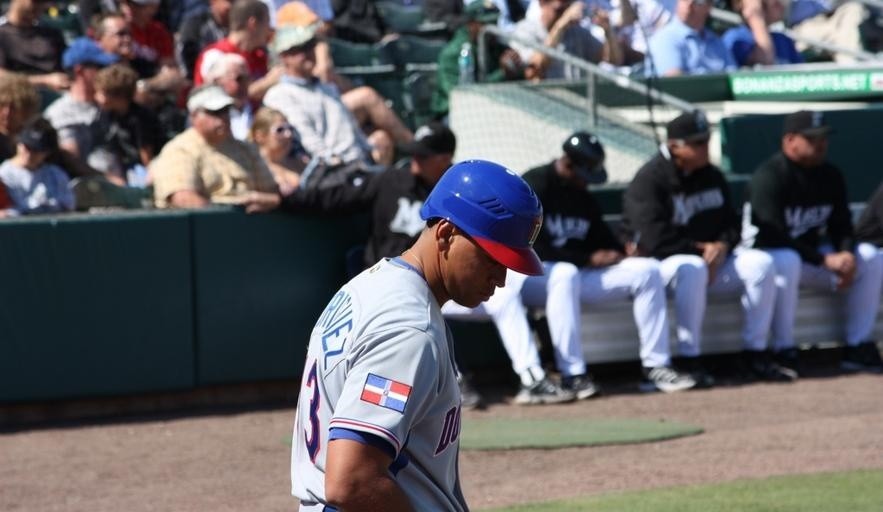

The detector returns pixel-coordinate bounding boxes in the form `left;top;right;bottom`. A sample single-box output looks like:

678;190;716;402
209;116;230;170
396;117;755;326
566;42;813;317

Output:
250;108;883;408
0;0;412;215
429;0;883;130
289;160;543;510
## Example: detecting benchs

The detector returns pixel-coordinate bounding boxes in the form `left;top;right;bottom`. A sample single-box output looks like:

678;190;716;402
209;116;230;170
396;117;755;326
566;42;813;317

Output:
440;262;882;394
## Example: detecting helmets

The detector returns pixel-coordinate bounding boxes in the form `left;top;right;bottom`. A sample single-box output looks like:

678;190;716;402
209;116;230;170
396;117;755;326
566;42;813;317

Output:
420;158;547;279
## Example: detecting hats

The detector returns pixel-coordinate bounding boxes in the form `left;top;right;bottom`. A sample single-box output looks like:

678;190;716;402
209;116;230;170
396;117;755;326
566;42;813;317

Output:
414;125;457;158
776;110;833;143
561;131;608;184
14;22;317;152
666;110;711;145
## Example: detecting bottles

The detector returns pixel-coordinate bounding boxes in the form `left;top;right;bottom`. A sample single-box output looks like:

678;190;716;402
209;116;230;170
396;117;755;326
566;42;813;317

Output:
457;42;475;87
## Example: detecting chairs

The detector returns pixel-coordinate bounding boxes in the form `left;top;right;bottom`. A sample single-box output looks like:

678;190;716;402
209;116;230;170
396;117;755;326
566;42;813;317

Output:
317;1;453;122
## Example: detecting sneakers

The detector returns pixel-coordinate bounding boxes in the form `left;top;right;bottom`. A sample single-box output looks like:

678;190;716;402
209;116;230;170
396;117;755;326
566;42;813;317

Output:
779;347;825;376
680;356;715;388
515;372;575;404
637;365;698;393
738;352;798;382
839;344;883;372
458;374;481;410
561;373;599;401
